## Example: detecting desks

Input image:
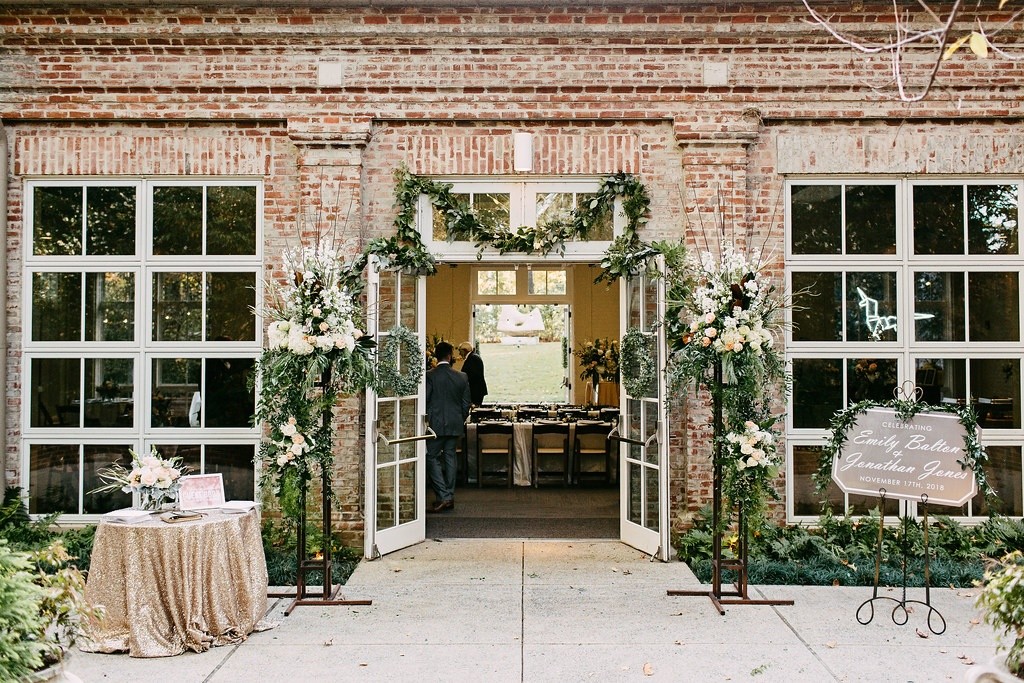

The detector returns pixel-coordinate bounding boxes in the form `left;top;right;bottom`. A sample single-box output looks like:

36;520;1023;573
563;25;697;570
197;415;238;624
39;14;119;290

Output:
472;408;620;419
74;502;279;657
468;421;618;484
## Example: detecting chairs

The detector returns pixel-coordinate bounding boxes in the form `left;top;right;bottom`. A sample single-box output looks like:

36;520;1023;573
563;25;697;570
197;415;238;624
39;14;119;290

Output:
556;411;589;423
521;405;545;410
516;410;549;423
556;404;583;410
476;423;514;490
530;423;571;491
598;408;620;423
470;410;502;422
455;423;470;488
480;404;513;410
572;424;613;487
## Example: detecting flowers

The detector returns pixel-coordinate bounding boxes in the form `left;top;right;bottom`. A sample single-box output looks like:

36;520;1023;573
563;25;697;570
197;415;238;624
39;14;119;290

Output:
647;166;819;533
243;165;427;533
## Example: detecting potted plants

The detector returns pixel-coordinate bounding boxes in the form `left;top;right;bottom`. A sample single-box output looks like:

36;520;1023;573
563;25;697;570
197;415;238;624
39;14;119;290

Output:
1;536;107;683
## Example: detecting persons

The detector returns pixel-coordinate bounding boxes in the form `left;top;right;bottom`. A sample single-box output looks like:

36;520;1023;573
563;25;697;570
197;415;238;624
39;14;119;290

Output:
426;342;469;513
457;341;488;408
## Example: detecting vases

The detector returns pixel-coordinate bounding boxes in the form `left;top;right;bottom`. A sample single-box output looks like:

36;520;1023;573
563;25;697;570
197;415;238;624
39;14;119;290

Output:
970;539;1024;682
85;445;195;512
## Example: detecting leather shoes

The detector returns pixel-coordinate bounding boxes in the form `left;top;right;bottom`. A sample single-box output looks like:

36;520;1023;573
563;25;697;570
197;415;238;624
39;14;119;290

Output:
427;501;457;515
